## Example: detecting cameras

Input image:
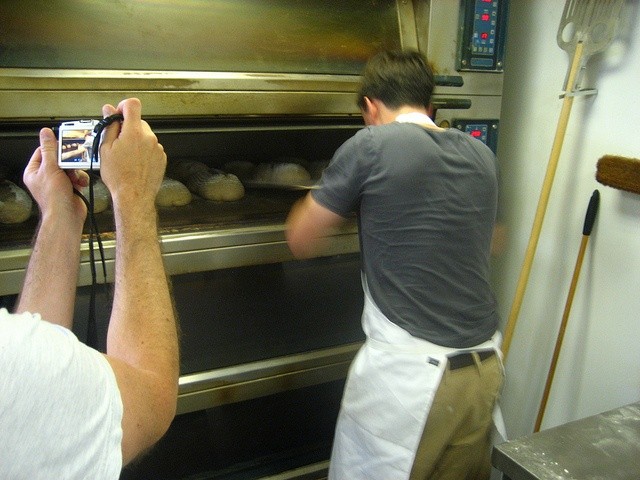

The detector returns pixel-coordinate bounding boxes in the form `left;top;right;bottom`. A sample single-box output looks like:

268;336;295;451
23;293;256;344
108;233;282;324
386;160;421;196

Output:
58;119;125;170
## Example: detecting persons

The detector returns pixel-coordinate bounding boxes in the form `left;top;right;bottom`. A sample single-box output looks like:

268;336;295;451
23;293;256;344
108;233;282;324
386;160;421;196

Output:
283;48;505;480
83;133;96;160
0;96;183;480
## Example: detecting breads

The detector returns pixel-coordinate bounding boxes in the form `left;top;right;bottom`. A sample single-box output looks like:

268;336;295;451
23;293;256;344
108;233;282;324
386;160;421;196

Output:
157;156;242;207
89;175;113;216
245;158;309;188
1;176;35;226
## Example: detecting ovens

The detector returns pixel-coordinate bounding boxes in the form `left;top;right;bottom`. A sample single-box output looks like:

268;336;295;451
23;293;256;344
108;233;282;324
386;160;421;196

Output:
0;0;509;480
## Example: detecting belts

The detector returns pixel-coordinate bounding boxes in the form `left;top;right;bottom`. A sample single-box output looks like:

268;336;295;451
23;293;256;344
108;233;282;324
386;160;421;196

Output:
448;350;495;370
